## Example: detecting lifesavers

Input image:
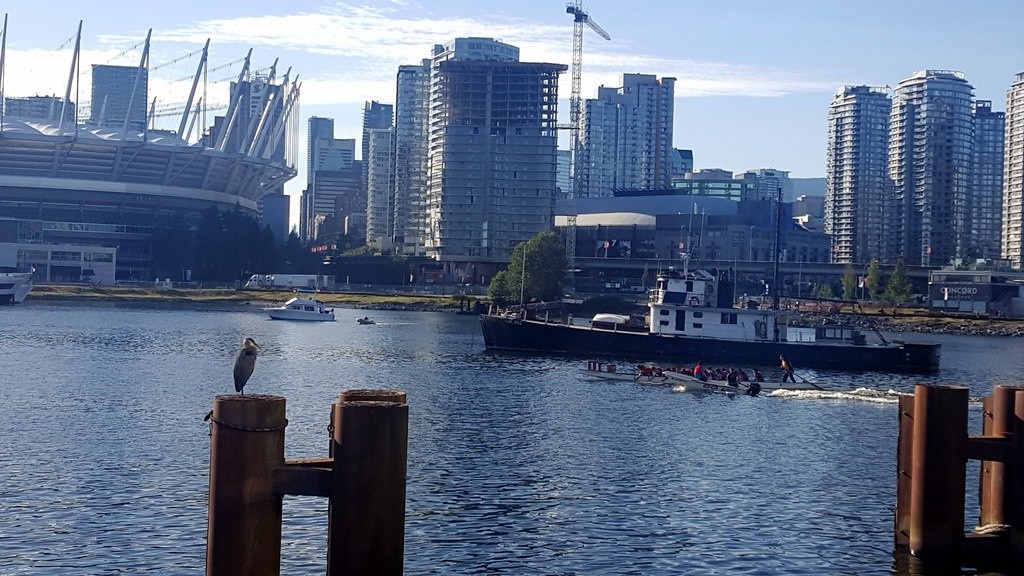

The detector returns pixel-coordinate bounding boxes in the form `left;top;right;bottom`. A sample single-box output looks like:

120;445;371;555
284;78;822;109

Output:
689;295;700;306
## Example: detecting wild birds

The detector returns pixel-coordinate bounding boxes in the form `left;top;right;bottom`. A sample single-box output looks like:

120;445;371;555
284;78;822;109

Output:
233;338;262;398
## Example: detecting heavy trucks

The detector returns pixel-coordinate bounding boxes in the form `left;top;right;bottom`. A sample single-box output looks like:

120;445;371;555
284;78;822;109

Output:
245;274;327;287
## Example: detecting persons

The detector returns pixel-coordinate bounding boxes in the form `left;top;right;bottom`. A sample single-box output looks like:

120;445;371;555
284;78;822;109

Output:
694;361;708;382
780;354;796;383
727;367;739;387
636;365;764;382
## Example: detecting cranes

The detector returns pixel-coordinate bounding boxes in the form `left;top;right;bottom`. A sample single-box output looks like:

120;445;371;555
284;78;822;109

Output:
564;0;611;149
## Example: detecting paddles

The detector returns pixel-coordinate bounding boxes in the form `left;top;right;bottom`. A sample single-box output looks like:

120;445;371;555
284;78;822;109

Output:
631;365;637;385
738;382;750;388
782;367;824;391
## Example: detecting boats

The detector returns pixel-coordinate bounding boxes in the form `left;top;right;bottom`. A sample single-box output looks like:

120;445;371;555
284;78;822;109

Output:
662;371;761;396
577;369;816;388
479;187;942;371
264;298;334;320
0;273;33;303
356;318;375;324
